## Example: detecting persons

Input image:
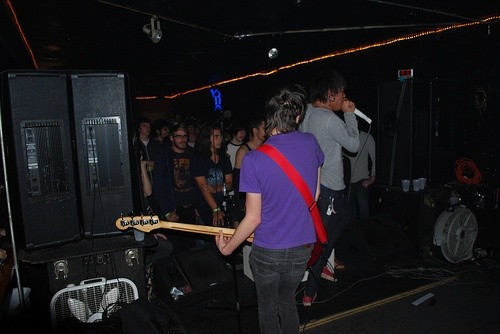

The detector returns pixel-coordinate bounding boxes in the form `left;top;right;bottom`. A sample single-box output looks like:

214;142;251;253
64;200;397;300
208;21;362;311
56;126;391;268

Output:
214;84;325;334
347;130;377;220
132;116;266;280
299;70;356;306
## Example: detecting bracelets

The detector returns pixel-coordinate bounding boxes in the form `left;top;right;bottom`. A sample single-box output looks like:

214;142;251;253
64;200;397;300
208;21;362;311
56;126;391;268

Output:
213;208;221;212
165;213;170;217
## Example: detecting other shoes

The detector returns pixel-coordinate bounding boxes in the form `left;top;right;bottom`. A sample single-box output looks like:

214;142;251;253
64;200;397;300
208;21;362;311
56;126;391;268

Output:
303;290;317;307
321;266;337;283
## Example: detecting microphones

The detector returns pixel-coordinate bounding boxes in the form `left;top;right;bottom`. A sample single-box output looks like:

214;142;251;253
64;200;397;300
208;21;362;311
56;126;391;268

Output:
354;109;372;124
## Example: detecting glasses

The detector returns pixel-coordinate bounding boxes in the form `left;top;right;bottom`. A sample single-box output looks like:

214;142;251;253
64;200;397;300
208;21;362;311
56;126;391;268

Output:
173;135;187;138
214;135;223;139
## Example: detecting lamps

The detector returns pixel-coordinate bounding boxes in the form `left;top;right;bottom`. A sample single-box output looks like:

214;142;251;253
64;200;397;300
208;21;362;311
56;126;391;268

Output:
142;15;163;44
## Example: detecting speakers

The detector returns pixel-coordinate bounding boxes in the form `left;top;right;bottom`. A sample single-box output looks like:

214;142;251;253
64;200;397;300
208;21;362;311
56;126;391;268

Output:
0;70;147;323
377;80;439;187
335;213;417;280
151;246;234;299
368;184;437;259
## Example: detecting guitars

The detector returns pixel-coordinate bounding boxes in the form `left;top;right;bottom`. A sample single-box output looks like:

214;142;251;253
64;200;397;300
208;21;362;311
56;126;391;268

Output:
116;209;322;268
